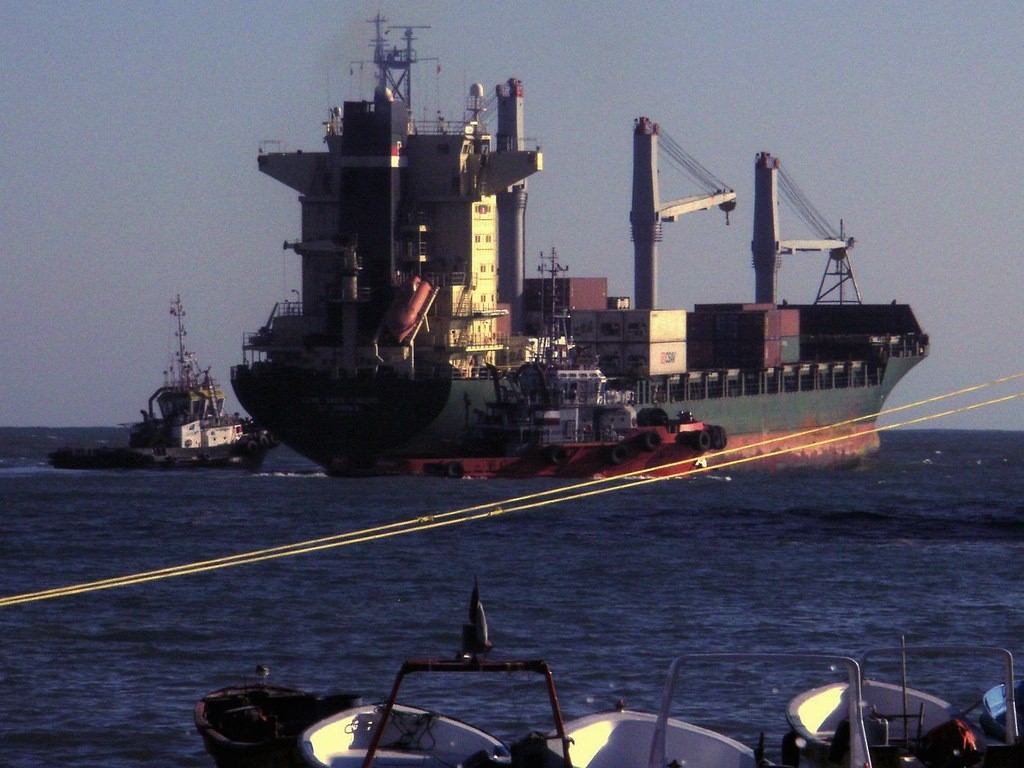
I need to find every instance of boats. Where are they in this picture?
[303,698,516,768]
[189,671,361,768]
[983,678,1024,745]
[550,701,781,768]
[784,675,987,754]
[369,252,726,480]
[54,291,280,474]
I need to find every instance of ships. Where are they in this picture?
[232,8,930,480]
